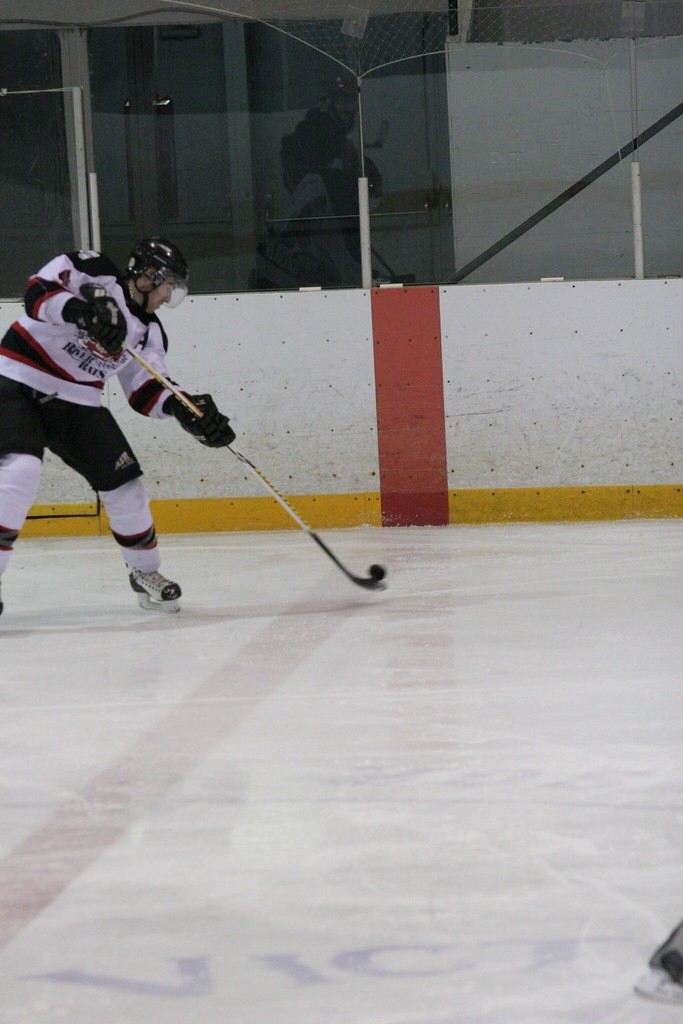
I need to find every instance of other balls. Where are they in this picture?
[369,564,385,577]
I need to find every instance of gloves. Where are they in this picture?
[162,391,236,447]
[62,296,127,355]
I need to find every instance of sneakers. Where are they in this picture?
[125,562,181,611]
[634,920,683,1006]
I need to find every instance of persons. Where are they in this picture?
[0,237,237,613]
[248,61,382,291]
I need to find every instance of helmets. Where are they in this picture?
[125,236,190,289]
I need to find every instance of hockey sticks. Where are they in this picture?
[89,314,385,588]
